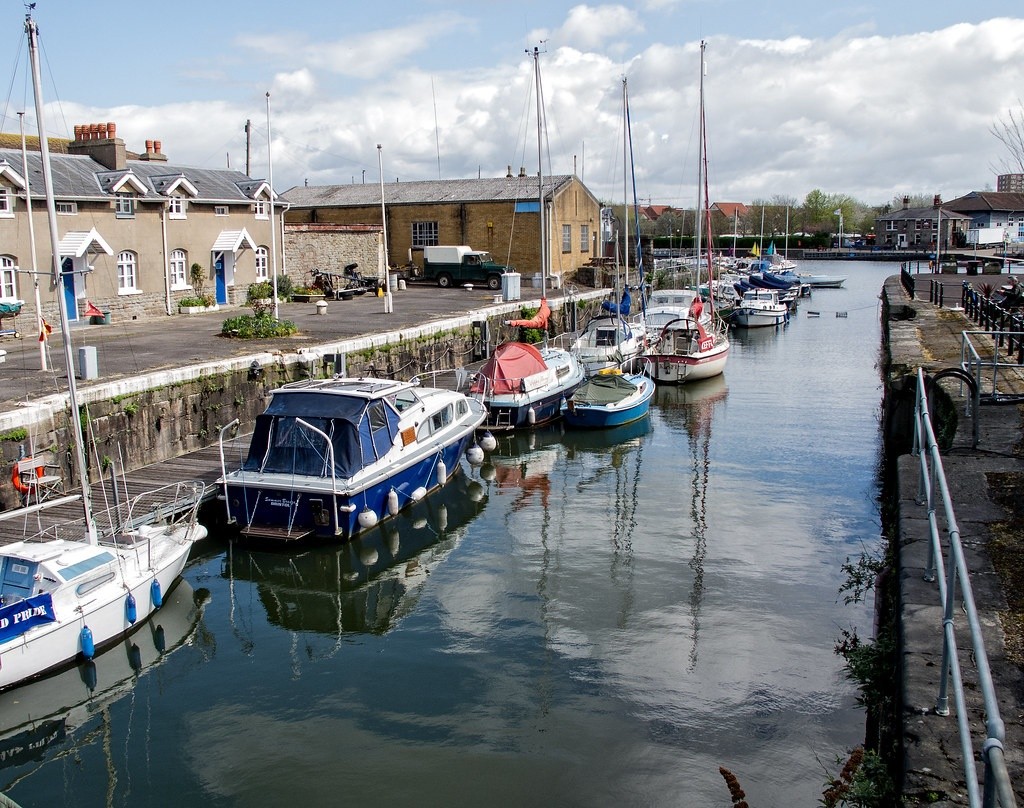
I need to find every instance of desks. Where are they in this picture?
[589,257,616,272]
[1001,285,1024,306]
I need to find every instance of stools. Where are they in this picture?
[583,263,598,268]
[603,262,620,270]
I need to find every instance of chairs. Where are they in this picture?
[17,455,67,508]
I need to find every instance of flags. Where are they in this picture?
[774,245,776,254]
[86,301,104,316]
[749,242,759,256]
[834,208,841,215]
[766,241,773,255]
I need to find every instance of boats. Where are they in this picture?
[560,356,658,427]
[632,287,711,337]
[695,252,848,329]
[211,374,490,544]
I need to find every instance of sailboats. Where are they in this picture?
[1,0,208,690]
[469,45,586,430]
[640,37,730,380]
[569,73,660,378]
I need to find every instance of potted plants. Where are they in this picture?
[248,274,293,305]
[179,263,220,314]
[291,285,326,303]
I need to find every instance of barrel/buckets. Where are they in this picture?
[95,311,111,325]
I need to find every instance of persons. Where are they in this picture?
[997,275,1024,312]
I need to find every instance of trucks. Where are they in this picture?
[423,246,516,290]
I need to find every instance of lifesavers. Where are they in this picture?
[11,457,45,494]
